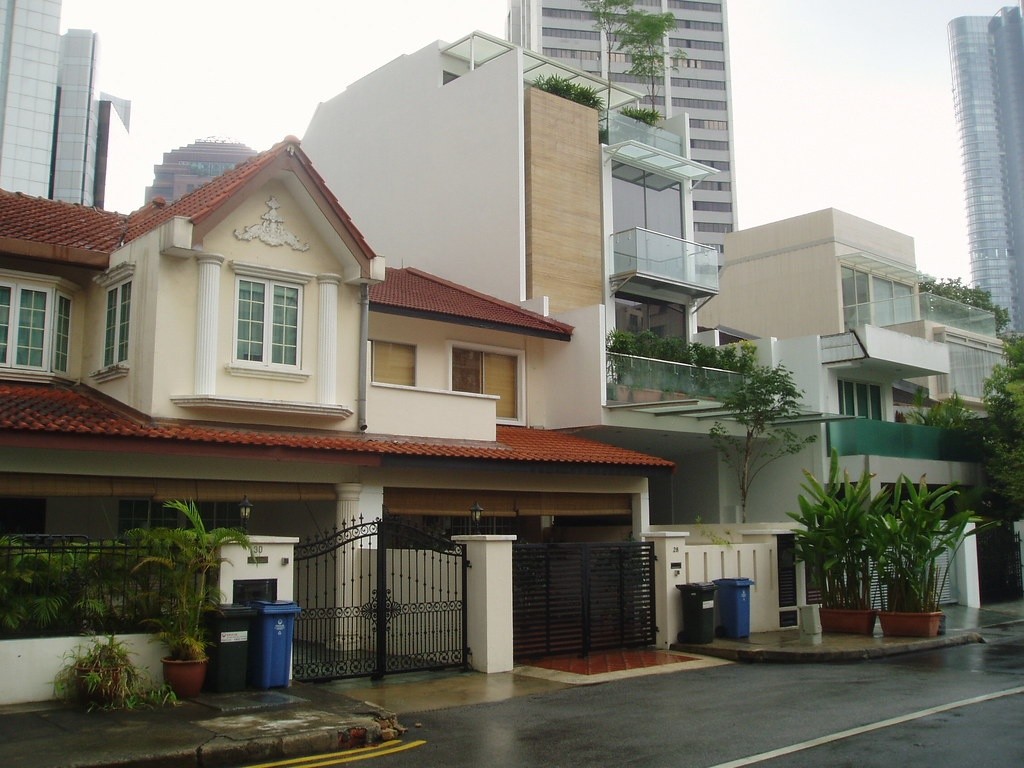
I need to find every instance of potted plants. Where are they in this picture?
[604,328,759,406]
[784,447,1005,638]
[130,498,256,697]
[55,627,187,712]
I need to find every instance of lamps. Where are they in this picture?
[469,500,484,534]
[238,494,254,533]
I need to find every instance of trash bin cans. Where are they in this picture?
[712,577,755,640]
[204,605,257,695]
[675,582,722,644]
[248,600,303,691]
